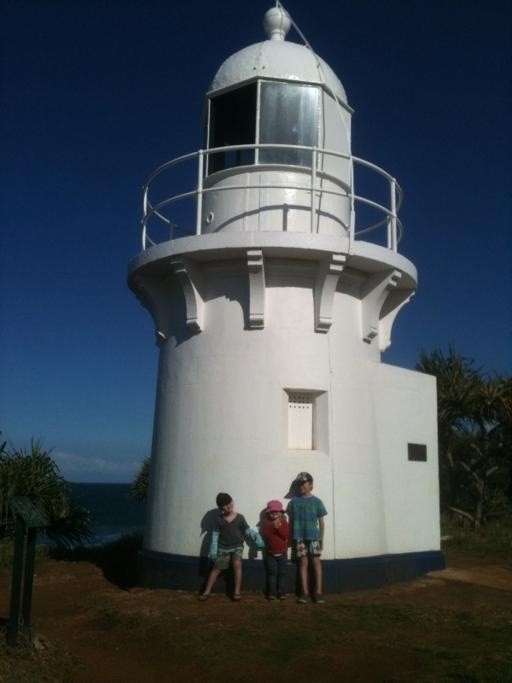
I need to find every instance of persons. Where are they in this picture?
[261,500,292,600]
[287,470,328,605]
[196,491,265,600]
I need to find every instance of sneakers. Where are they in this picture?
[268,595,286,600]
[297,593,325,604]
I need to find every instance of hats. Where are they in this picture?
[217,493,231,506]
[295,472,313,483]
[265,500,286,512]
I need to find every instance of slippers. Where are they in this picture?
[199,593,209,601]
[233,593,241,600]
